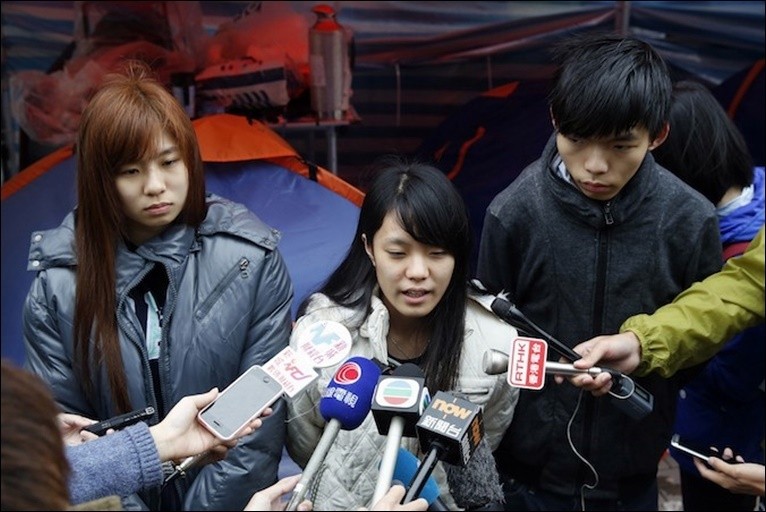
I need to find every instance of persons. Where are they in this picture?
[554,224,766,512]
[473,28,723,512]
[285,162,521,511]
[649,80,766,512]
[21,61,293,512]
[0,357,429,512]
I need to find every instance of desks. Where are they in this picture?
[266,101,362,177]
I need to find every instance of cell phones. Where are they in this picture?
[79,406,155,442]
[669,433,743,465]
[197,364,285,441]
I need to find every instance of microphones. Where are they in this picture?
[491,297,653,421]
[482,337,623,390]
[159,321,485,512]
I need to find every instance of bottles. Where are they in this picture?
[307,5,345,121]
